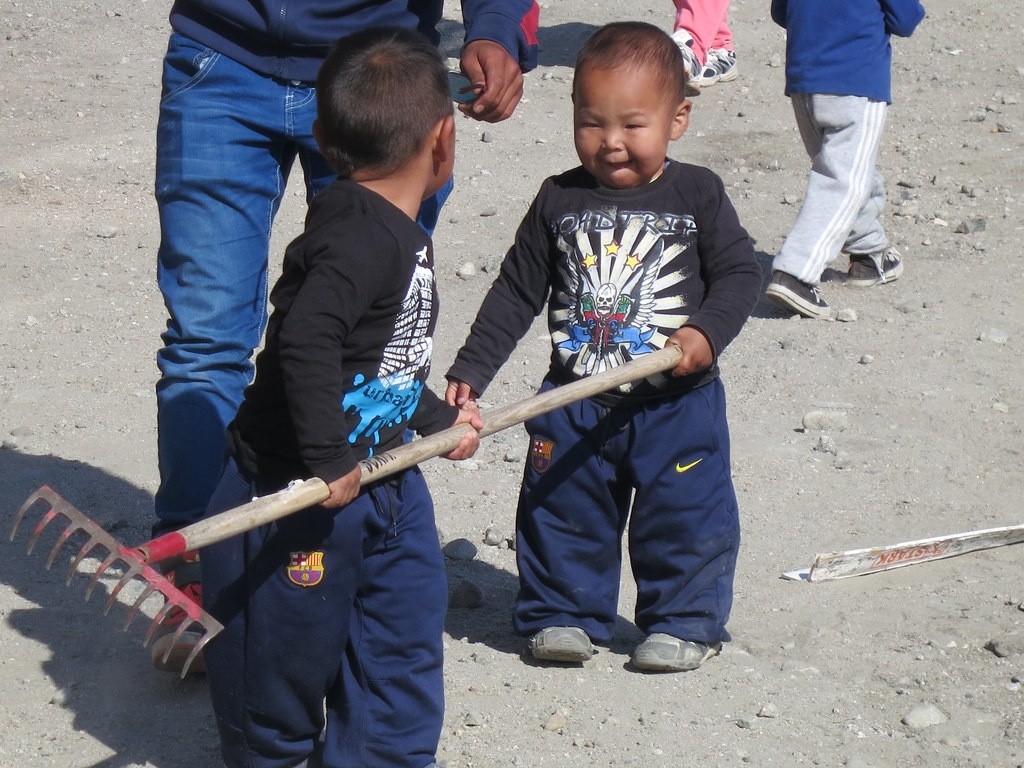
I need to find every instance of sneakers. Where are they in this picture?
[766,270,831,318]
[150,625,204,671]
[632,632,723,673]
[845,254,904,289]
[529,626,592,662]
[673,41,739,86]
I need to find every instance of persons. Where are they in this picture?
[661,0,741,88]
[194,23,480,768]
[767,0,928,322]
[439,21,765,671]
[152,0,539,676]
